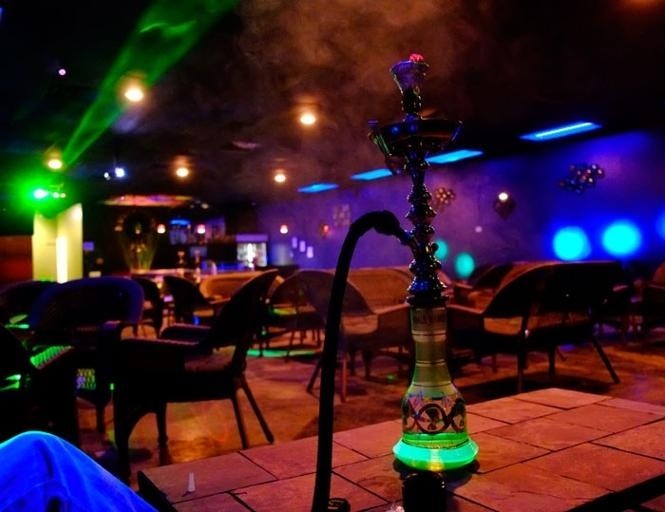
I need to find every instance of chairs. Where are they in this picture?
[3,278,58,338]
[445,259,625,397]
[30,274,144,437]
[270,270,323,348]
[198,272,286,305]
[0,326,75,445]
[109,268,276,462]
[293,268,416,402]
[163,275,229,321]
[348,268,416,380]
[471,259,559,376]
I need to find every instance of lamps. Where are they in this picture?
[303,53,482,512]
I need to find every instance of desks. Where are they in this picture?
[133,386,665,510]
